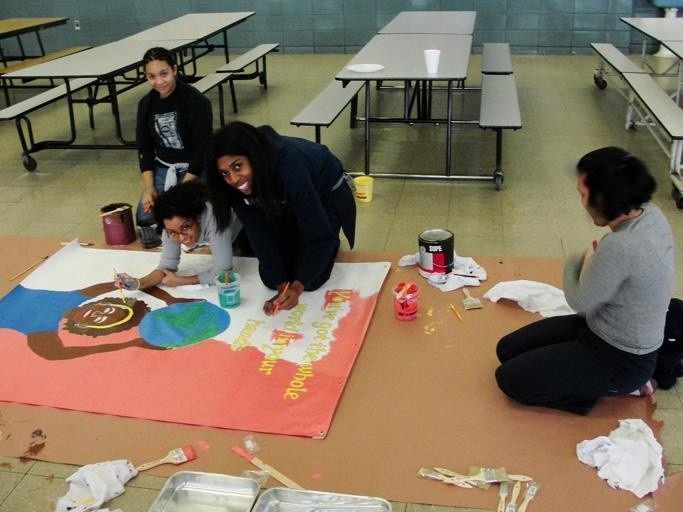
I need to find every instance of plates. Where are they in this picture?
[346,64,385,75]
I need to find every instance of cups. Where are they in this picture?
[424,49,441,74]
[391,283,419,321]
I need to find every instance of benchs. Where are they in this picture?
[290,76,366,145]
[1,11,280,171]
[621,72,683,177]
[475,74,522,190]
[481,41,513,75]
[589,41,649,132]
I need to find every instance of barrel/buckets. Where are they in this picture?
[353,176,374,203]
[392,278,420,320]
[101,202,137,246]
[419,228,454,282]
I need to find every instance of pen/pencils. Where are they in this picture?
[144,192,158,214]
[450,303,464,322]
[272,281,290,318]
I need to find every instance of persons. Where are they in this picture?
[208,121,356,317]
[499,147,676,417]
[136,46,212,228]
[112,183,242,291]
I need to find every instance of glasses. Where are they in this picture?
[166,217,194,239]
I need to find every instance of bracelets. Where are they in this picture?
[135,277,141,292]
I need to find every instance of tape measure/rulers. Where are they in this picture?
[230,445,305,490]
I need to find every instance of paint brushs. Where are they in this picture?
[113,268,127,305]
[10,256,49,281]
[60,242,95,246]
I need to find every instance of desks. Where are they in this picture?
[335,33,473,177]
[375,11,477,122]
[618,15,683,107]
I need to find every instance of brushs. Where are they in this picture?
[462,288,482,310]
[137,445,197,471]
[100,205,130,217]
[416,467,541,512]
